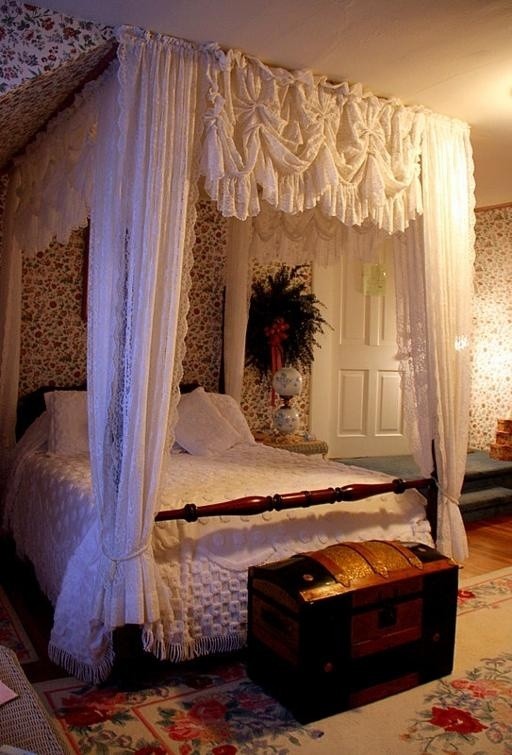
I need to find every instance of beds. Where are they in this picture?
[0,385,465,689]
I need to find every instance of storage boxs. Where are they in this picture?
[247,540,464,723]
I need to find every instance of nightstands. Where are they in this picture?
[252,432,328,460]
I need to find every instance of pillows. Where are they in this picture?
[43,387,252,458]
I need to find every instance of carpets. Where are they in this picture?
[33,565,512,755]
[0,590,38,664]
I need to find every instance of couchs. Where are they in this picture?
[0,644,77,755]
